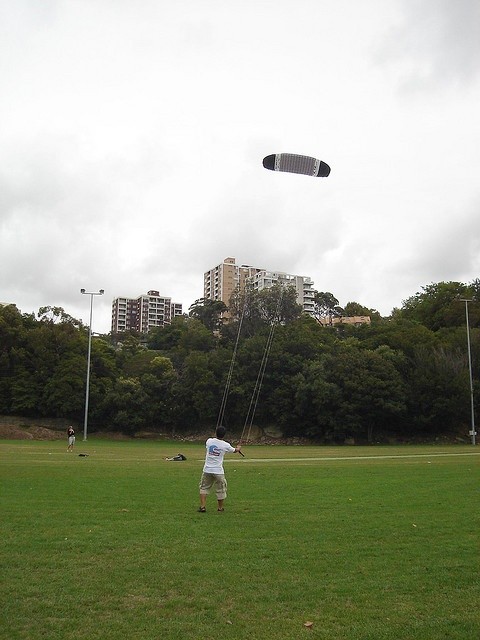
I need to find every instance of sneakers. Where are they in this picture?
[67,450,68,453]
[71,450,72,452]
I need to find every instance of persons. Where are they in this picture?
[165,454,185,460]
[66,426,75,452]
[199,426,240,513]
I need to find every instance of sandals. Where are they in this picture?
[197,507,206,512]
[218,508,224,511]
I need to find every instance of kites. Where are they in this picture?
[263,153,331,178]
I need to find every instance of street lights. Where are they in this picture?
[458,295,477,445]
[81,289,104,440]
